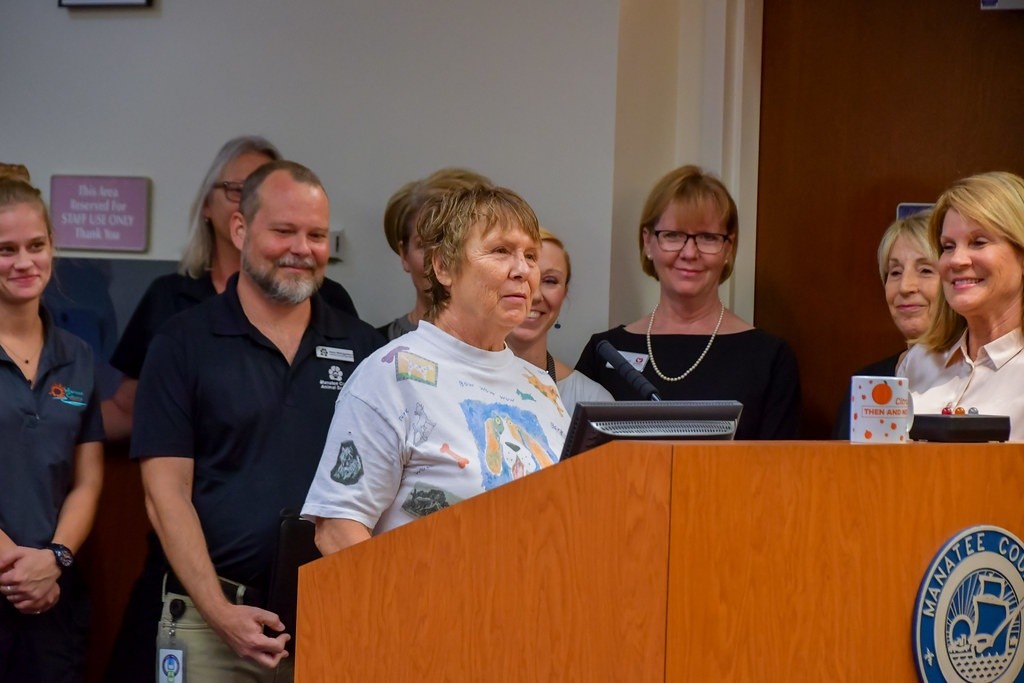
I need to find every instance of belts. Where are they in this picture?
[166,573,248,609]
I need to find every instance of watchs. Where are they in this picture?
[47,543,73,567]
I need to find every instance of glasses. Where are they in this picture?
[651,229,730,254]
[212,179,241,201]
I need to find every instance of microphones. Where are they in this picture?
[597,340,661,401]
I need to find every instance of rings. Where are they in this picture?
[7,586,10,591]
[35,611,40,614]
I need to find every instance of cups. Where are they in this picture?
[851,375,914,444]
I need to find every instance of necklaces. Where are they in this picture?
[646,303,723,381]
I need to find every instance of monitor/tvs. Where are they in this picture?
[559,400,743,462]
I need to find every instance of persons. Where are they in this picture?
[0,135,1024,683]
[575,167,797,441]
